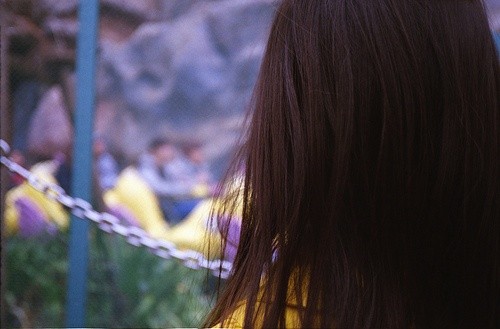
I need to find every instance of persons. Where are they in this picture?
[195,2,500,329]
[1,0,221,211]
